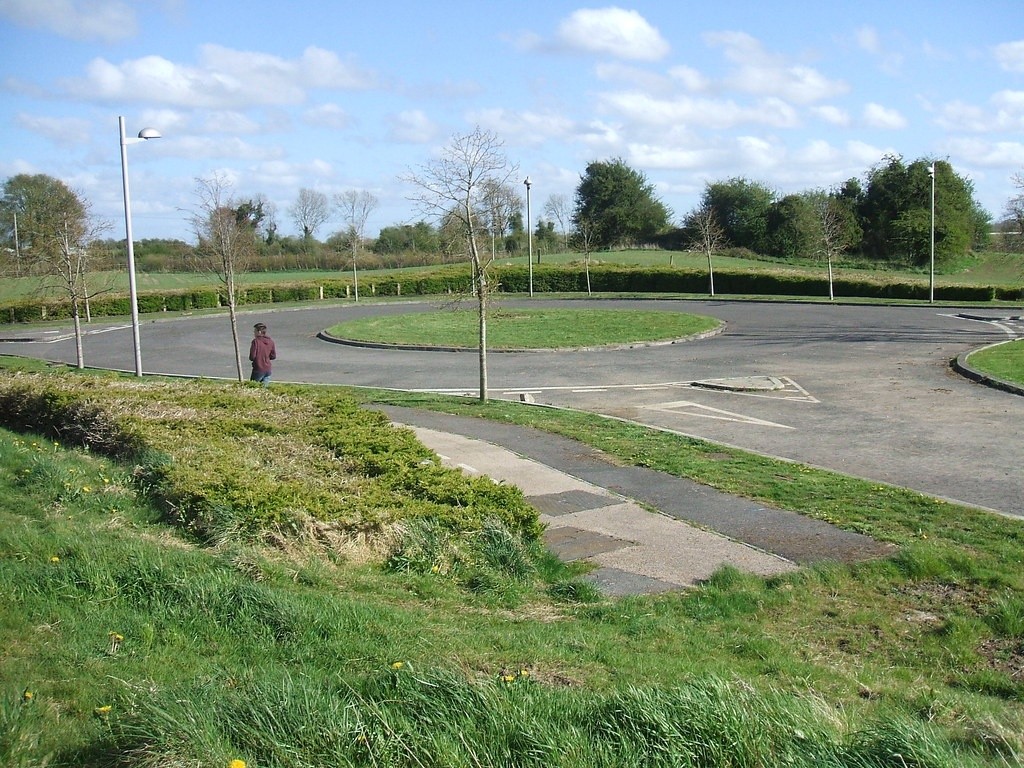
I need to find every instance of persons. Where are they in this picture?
[248,322,277,388]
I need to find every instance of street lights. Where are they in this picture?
[523,175,534,298]
[926,163,937,303]
[118,116,163,376]
